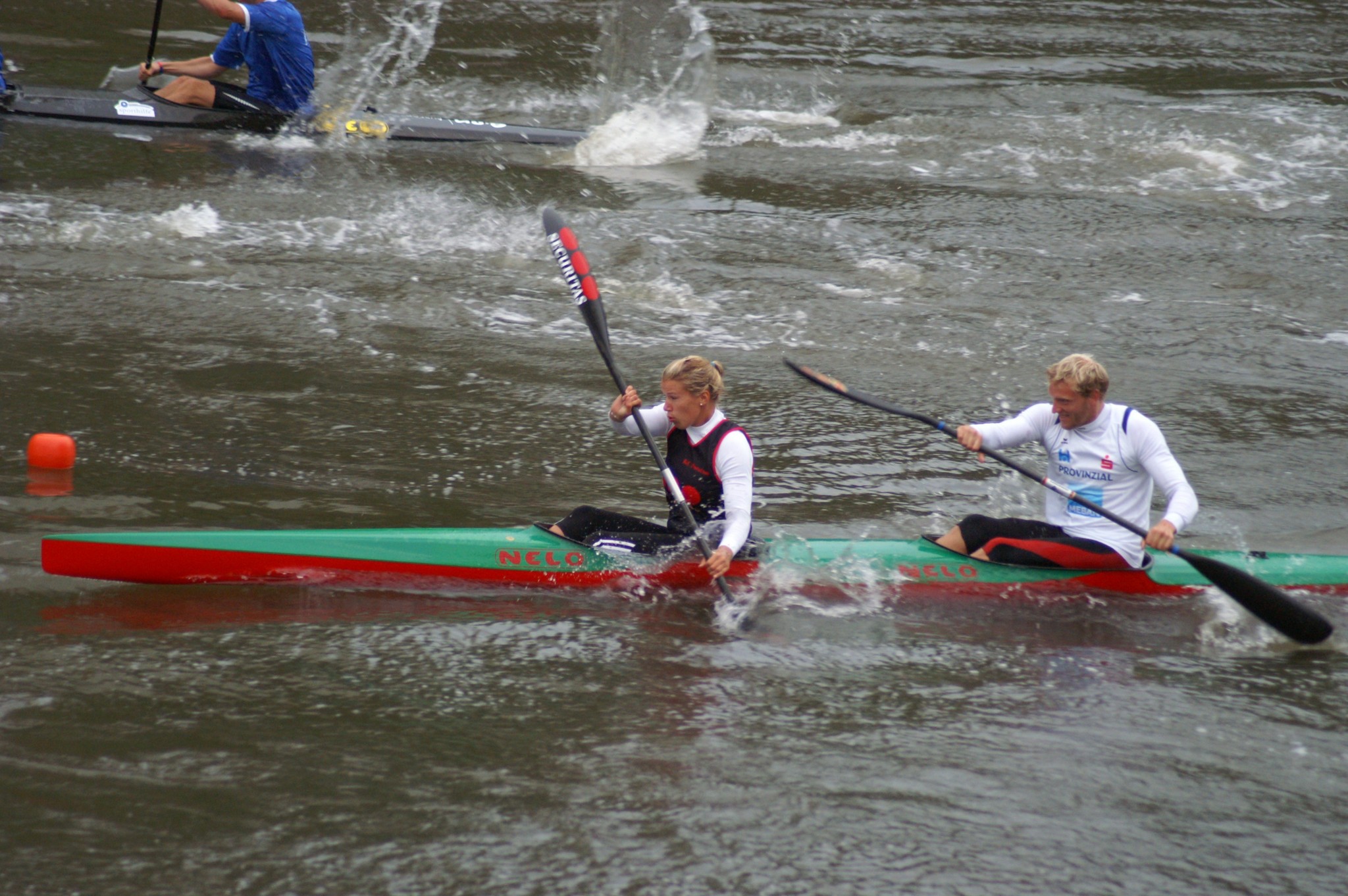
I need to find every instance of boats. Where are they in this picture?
[0,68,592,146]
[42,520,1348,607]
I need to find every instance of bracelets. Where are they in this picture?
[156,60,164,73]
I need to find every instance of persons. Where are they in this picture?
[138,1,316,116]
[548,356,753,579]
[936,354,1198,570]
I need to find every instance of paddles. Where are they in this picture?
[141,0,164,87]
[540,207,736,604]
[782,355,1336,646]
